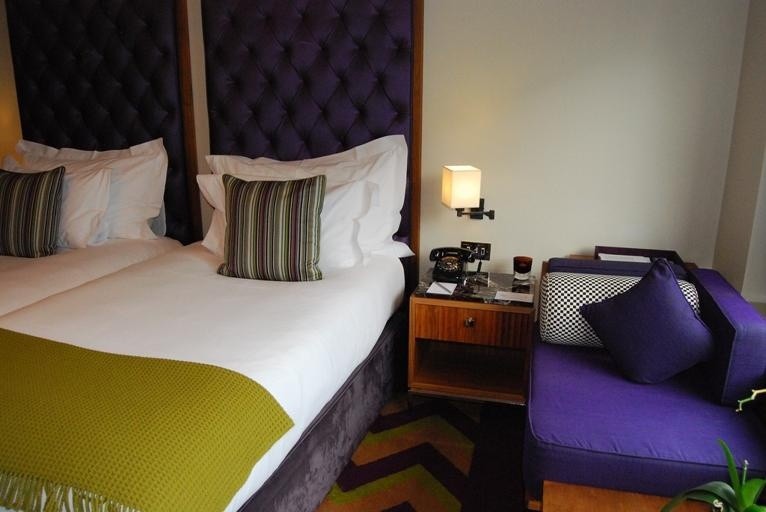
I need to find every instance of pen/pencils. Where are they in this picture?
[435,280,452,294]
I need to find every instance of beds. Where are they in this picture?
[0,0,425,512]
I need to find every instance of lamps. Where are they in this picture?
[441,164,496,222]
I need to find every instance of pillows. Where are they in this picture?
[580,258,721,386]
[0,166,66,258]
[539,269,702,347]
[15,137,168,240]
[205,134,417,258]
[216,174,326,282]
[2,155,118,249]
[195,174,370,272]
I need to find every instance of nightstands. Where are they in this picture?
[407,268,536,406]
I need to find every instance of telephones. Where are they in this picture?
[430,248,476,285]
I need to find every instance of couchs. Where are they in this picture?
[523,258,766,512]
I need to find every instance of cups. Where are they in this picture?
[514,256,533,281]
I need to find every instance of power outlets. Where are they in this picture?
[461,241,491,261]
